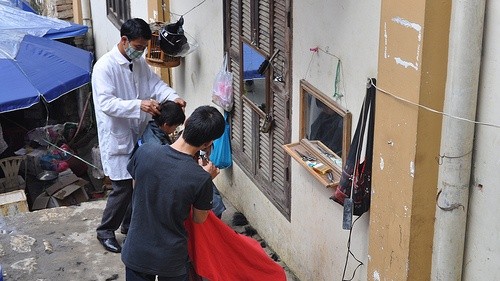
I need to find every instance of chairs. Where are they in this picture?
[0,156,27,193]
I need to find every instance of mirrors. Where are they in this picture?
[299,79,352,177]
[238,35,271,121]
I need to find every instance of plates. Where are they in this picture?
[36,171,59,181]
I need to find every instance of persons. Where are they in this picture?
[92,18,187,252]
[310,98,343,158]
[121,100,226,281]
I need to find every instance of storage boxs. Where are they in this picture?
[32,173,90,208]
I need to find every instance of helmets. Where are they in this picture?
[159,17,190,56]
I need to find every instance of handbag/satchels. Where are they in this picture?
[209,110,232,169]
[212,52,233,112]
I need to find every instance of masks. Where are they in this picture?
[123,43,143,60]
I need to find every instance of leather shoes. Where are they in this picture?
[97,235,122,253]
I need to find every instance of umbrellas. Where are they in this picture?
[0,0,94,114]
